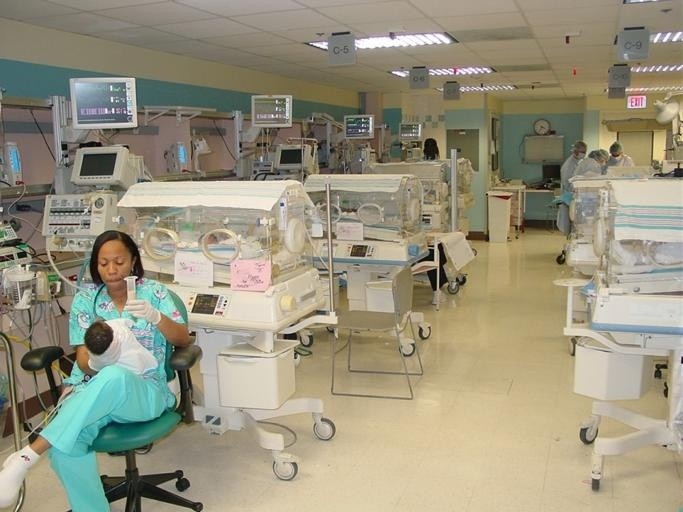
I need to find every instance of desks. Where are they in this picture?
[525,189,554,221]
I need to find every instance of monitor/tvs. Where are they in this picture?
[543,164,560,188]
[344,114,375,140]
[70,147,137,192]
[69,77,138,130]
[251,94,293,128]
[275,144,314,175]
[400,122,422,138]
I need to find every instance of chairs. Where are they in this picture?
[331,268,424,401]
[18,289,204,512]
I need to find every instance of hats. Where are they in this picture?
[609,142,622,153]
[596,150,610,161]
[574,141,586,150]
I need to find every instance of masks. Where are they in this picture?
[599,161,606,167]
[574,153,586,160]
[612,155,622,161]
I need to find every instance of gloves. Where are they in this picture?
[87,338,121,371]
[124,299,161,325]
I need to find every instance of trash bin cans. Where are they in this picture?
[486,191,513,243]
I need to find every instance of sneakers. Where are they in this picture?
[0,451,29,508]
[440,283,450,295]
[433,291,442,306]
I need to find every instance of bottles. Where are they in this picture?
[123,276,138,300]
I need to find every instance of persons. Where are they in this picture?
[412,242,451,303]
[84,319,113,355]
[0,232,189,512]
[611,143,633,168]
[424,137,440,159]
[554,141,587,237]
[573,149,609,177]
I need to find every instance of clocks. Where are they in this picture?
[533,119,551,136]
